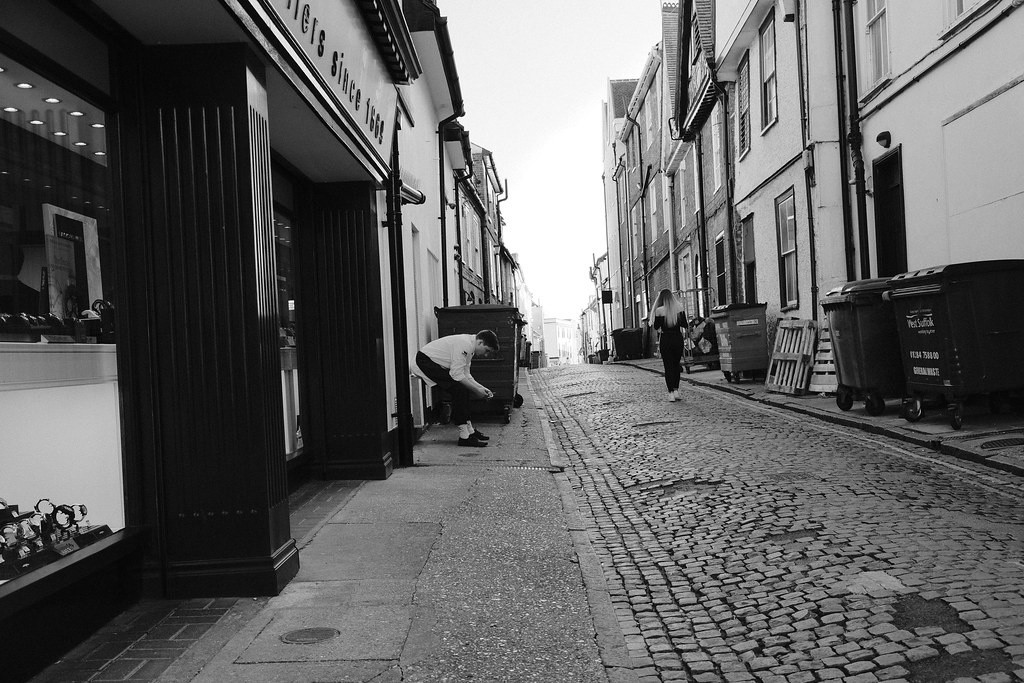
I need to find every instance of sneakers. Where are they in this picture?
[668,393,675,401]
[674,390,680,399]
[470,428,489,440]
[458,435,488,447]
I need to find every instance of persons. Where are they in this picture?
[648,289,688,401]
[415,330,499,447]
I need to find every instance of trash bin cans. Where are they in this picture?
[818,276,903,417]
[610,328,643,362]
[882,260,1024,424]
[433,305,532,424]
[710,301,769,382]
